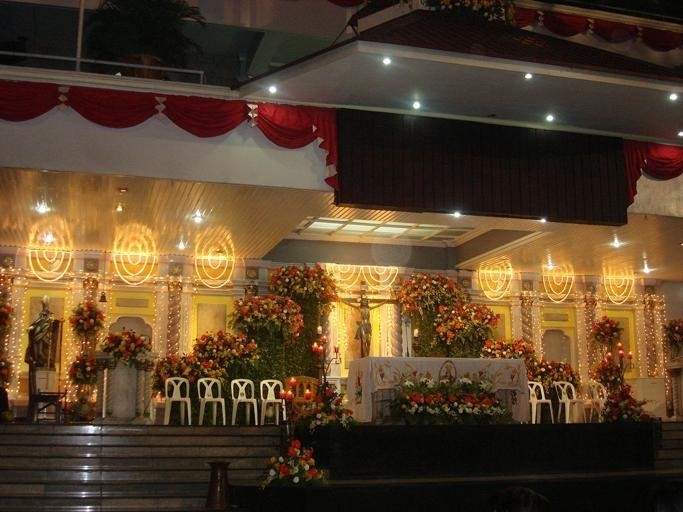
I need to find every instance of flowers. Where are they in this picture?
[261,434,335,491]
[156,262,351,431]
[395,273,582,388]
[67,298,151,386]
[591,315,654,422]
[398,374,506,426]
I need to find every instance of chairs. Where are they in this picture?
[26,358,66,423]
[525,376,608,424]
